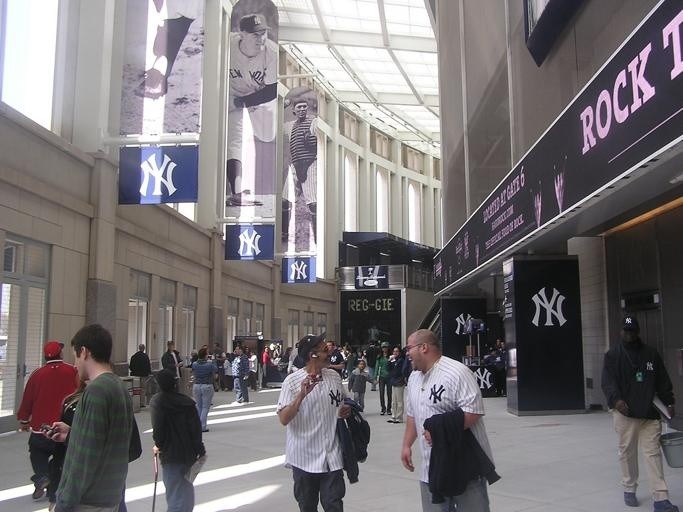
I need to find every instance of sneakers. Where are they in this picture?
[279,231,291,242]
[387,417,404,424]
[29,472,51,498]
[653,499,680,512]
[380,410,386,415]
[622,490,639,507]
[387,411,392,415]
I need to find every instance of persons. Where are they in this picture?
[150,369,204,512]
[19,324,133,511]
[401,329,496,512]
[129,344,151,407]
[261,342,415,423]
[133,0,205,98]
[277,332,352,512]
[161,341,258,403]
[192,347,216,433]
[226,13,279,206]
[290,100,318,184]
[485,338,506,396]
[602,315,680,512]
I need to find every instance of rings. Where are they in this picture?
[347,413,349,416]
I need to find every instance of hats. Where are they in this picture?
[619,316,642,331]
[237,12,274,33]
[44,342,64,357]
[381,341,389,348]
[297,331,328,360]
[157,369,180,392]
[292,97,309,108]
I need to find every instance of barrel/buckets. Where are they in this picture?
[659,418,683,468]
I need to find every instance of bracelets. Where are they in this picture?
[667,405,675,408]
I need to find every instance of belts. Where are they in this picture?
[195,382,212,385]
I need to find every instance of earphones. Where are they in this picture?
[312,354,319,358]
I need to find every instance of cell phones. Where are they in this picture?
[40,424,58,436]
